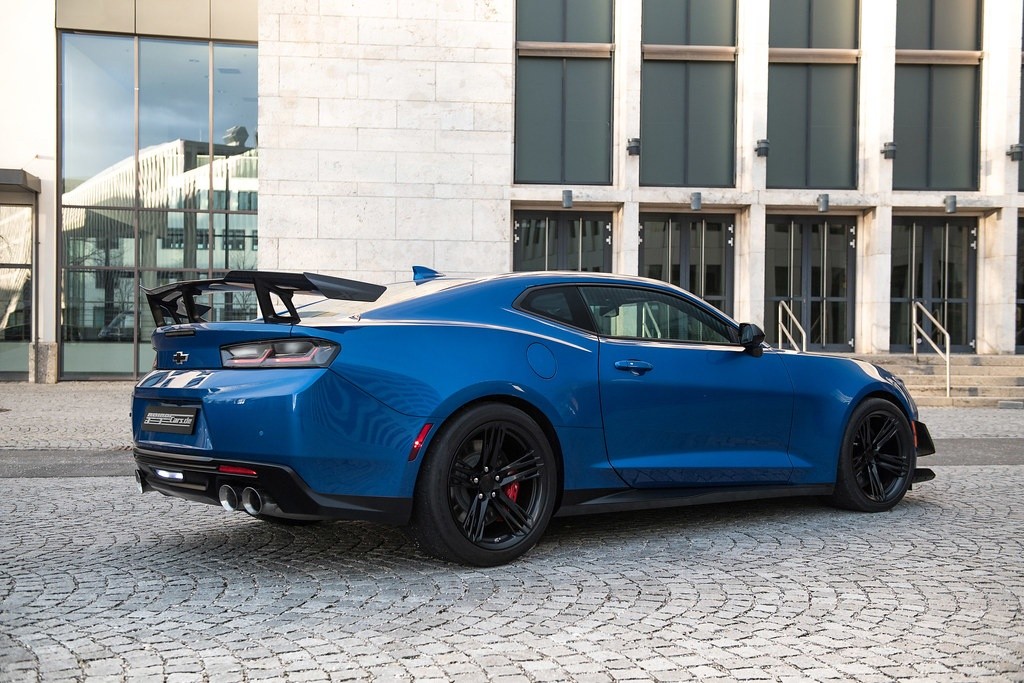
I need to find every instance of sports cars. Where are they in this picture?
[127,264,939,567]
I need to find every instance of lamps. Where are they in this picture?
[816,193,830,212]
[755,139,769,158]
[691,192,701,211]
[562,190,573,209]
[1007,144,1023,160]
[944,196,958,215]
[881,141,897,159]
[628,137,641,157]
